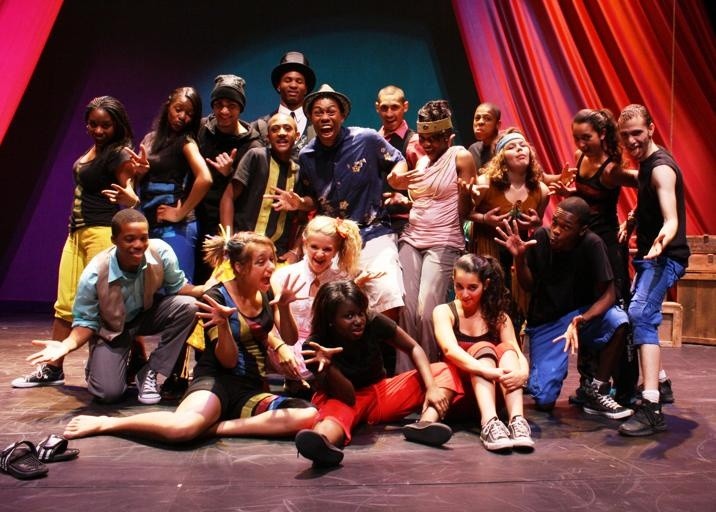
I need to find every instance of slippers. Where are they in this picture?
[0,440,49,478]
[35,433,81,463]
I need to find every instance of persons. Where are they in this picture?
[432,252,536,452]
[132,86,215,397]
[548,105,644,410]
[23,206,224,406]
[493,196,635,420]
[466,102,502,175]
[617,103,693,437]
[179,73,261,286]
[395,99,478,375]
[269,214,387,401]
[220,109,310,262]
[10,95,142,388]
[294,280,467,466]
[249,50,317,148]
[461,127,550,351]
[298,82,425,327]
[62,230,322,443]
[374,84,427,171]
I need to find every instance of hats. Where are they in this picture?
[302,83,352,121]
[210,74,248,114]
[271,51,317,96]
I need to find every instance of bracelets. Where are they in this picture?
[272,341,288,354]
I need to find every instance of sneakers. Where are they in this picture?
[12,363,65,388]
[295,429,344,464]
[402,420,453,447]
[480,376,675,451]
[134,360,162,405]
